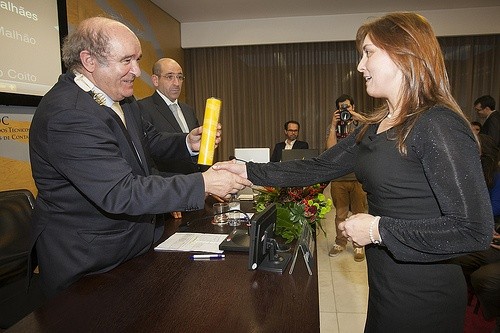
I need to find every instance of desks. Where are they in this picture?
[3,186,320,333]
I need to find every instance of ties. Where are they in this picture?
[169,104,186,132]
[111,102,127,129]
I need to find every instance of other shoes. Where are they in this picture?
[354,247,365,263]
[329,243,344,257]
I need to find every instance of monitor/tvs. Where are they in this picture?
[282,149,320,162]
[248,203,293,272]
[234,148,270,163]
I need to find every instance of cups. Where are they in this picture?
[213,203,228,223]
[227,202,241,227]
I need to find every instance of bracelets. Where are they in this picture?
[369,215,381,244]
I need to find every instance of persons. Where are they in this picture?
[26,17,255,289]
[270,121,308,162]
[213,12,495,333]
[137,58,206,218]
[470,95,500,318]
[326,94,369,263]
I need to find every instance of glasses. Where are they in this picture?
[154,74,186,83]
[286,130,298,132]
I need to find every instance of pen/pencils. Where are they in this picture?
[189,253,226,259]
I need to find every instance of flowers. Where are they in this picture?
[250,181,334,245]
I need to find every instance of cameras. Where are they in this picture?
[336,104,353,125]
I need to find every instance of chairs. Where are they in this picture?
[0,188,36,333]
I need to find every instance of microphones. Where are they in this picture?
[229,155,247,163]
[178,209,251,251]
